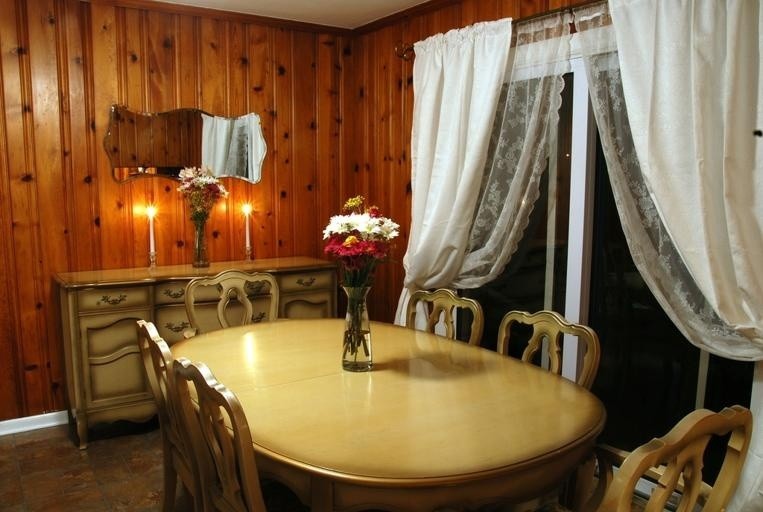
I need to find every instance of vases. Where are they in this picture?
[191,222,211,267]
[341,287,373,373]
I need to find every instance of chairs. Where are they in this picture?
[171,356,313,512]
[184,269,280,336]
[496,309,602,512]
[406,287,486,345]
[536,404,754,511]
[134,319,204,512]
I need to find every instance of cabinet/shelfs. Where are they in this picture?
[53,254,341,451]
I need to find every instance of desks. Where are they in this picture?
[166,319,609,512]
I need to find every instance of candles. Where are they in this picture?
[245,213,250,248]
[148,208,155,253]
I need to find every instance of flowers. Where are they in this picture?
[322,195,401,363]
[176,167,230,261]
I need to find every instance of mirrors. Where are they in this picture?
[103,102,268,185]
